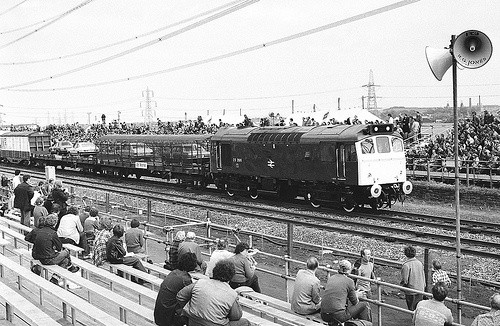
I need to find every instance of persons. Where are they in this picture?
[471,293,500,326]
[413,282,453,326]
[163,231,207,275]
[154,252,197,326]
[400,246,426,319]
[404,110,500,178]
[205,237,267,306]
[350,248,377,309]
[291,257,322,323]
[0,167,153,287]
[432,260,451,303]
[321,260,369,326]
[0,111,424,157]
[176,261,252,326]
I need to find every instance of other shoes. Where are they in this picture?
[255,299,268,305]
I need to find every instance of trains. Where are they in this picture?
[0,123,413,213]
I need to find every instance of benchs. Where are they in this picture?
[0,211,329,326]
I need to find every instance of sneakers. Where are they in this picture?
[48,268,82,288]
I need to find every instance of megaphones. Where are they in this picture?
[452,30,493,69]
[425,46,454,81]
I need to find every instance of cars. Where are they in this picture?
[161,143,210,164]
[115,142,153,162]
[49,141,99,157]
